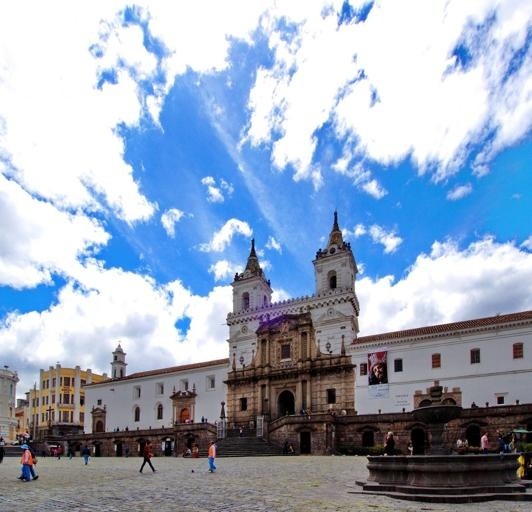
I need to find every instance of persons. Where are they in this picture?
[81,445,91,465]
[288,444,294,455]
[385,432,395,455]
[18,435,30,445]
[481,432,489,454]
[208,441,216,472]
[126,446,129,458]
[368,363,388,384]
[184,447,191,455]
[496,432,519,452]
[58,445,63,459]
[193,446,198,458]
[457,435,468,448]
[18,444,38,482]
[140,442,157,472]
[70,446,73,458]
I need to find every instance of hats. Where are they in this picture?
[20,443,29,450]
